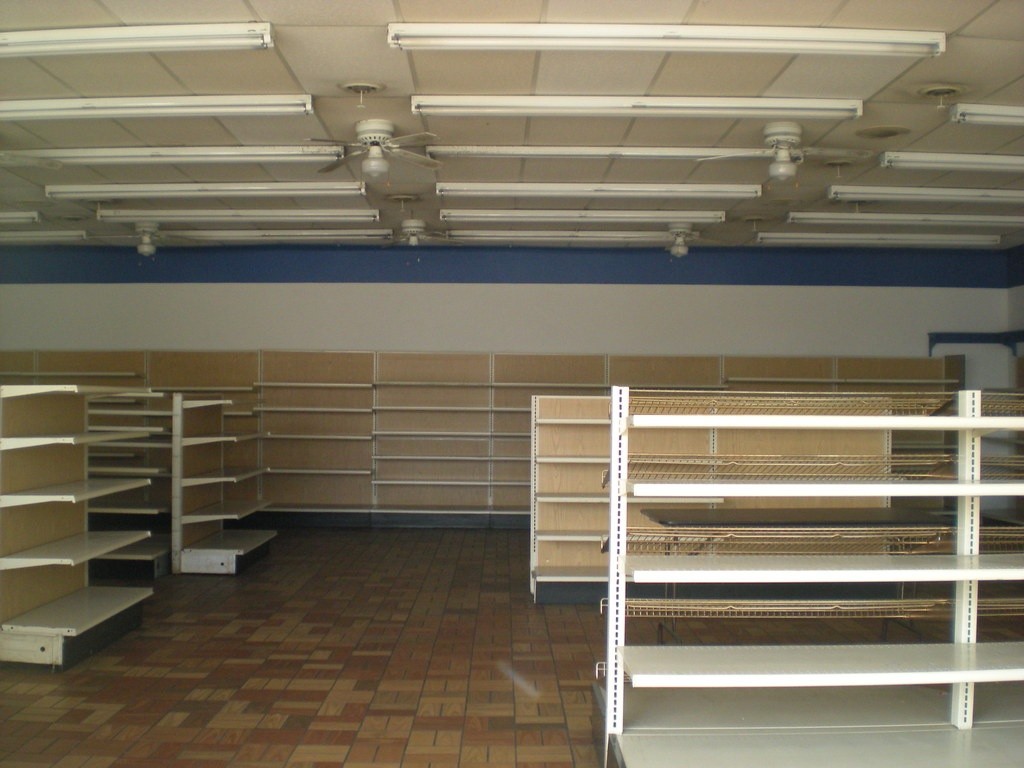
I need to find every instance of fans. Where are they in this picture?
[95,225,194,247]
[300,119,444,176]
[693,121,875,170]
[380,219,451,249]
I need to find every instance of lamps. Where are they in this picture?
[45,182,368,202]
[0,212,45,227]
[408,234,419,246]
[164,229,395,244]
[0,93,315,122]
[0,230,91,246]
[666,222,694,258]
[447,230,702,245]
[0,144,347,170]
[755,102,1024,247]
[361,144,389,178]
[138,237,155,256]
[411,93,862,120]
[438,210,726,224]
[387,22,949,60]
[437,182,764,201]
[0,22,277,59]
[96,209,381,223]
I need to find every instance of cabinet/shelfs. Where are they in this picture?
[87,390,171,585]
[145,348,260,525]
[171,393,275,575]
[491,353,607,529]
[253,350,373,531]
[722,355,836,394]
[36,350,147,393]
[596,386,1024,768]
[605,355,721,390]
[836,354,965,514]
[529,394,894,605]
[0,386,153,671]
[368,353,491,529]
